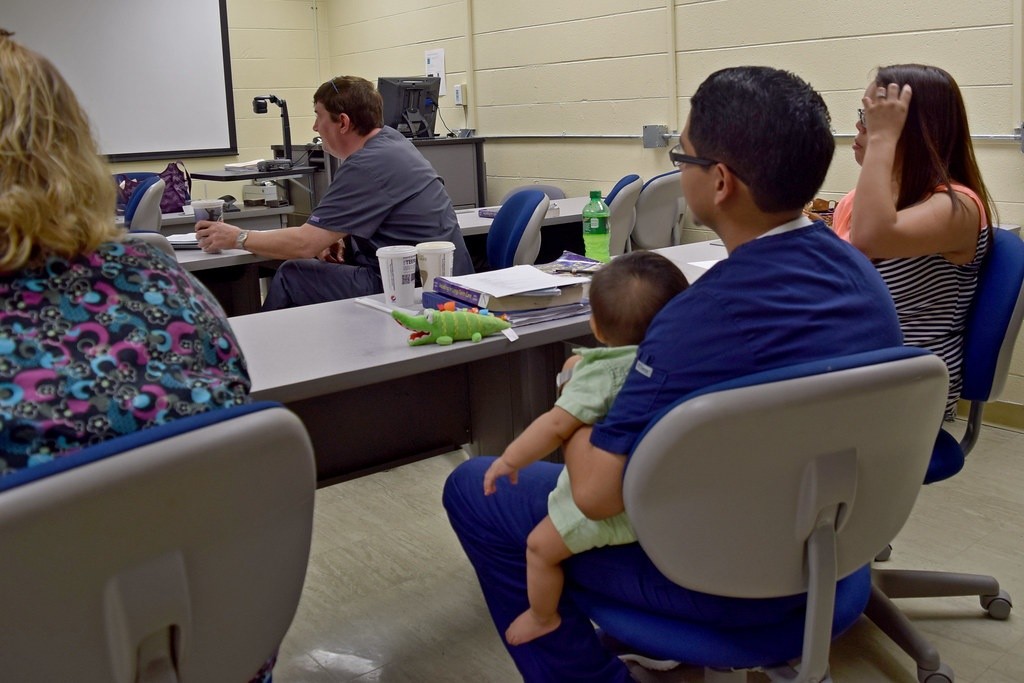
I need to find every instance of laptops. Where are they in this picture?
[166,234,200,249]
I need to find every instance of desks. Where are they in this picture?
[228,236,753,403]
[118,201,295,235]
[169,193,657,275]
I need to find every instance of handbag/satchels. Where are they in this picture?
[115,161,191,215]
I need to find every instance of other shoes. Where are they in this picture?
[593,627,679,670]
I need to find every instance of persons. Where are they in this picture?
[194,76,476,312]
[442,66,904,683]
[833,64,995,423]
[482,249,690,646]
[0,36,252,477]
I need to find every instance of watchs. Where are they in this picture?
[238,230,251,250]
[556,368,572,400]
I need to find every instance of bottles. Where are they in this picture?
[581,190,611,263]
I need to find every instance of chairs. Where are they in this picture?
[0,400,316,683]
[864,225,1024,683]
[585,347,948,683]
[124,175,165,230]
[486,170,687,269]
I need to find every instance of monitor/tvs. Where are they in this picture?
[378,77,441,138]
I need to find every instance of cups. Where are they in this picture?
[415,240,457,293]
[190,199,225,254]
[376,246,418,308]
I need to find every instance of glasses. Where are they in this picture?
[668,143,753,188]
[858,108,866,129]
